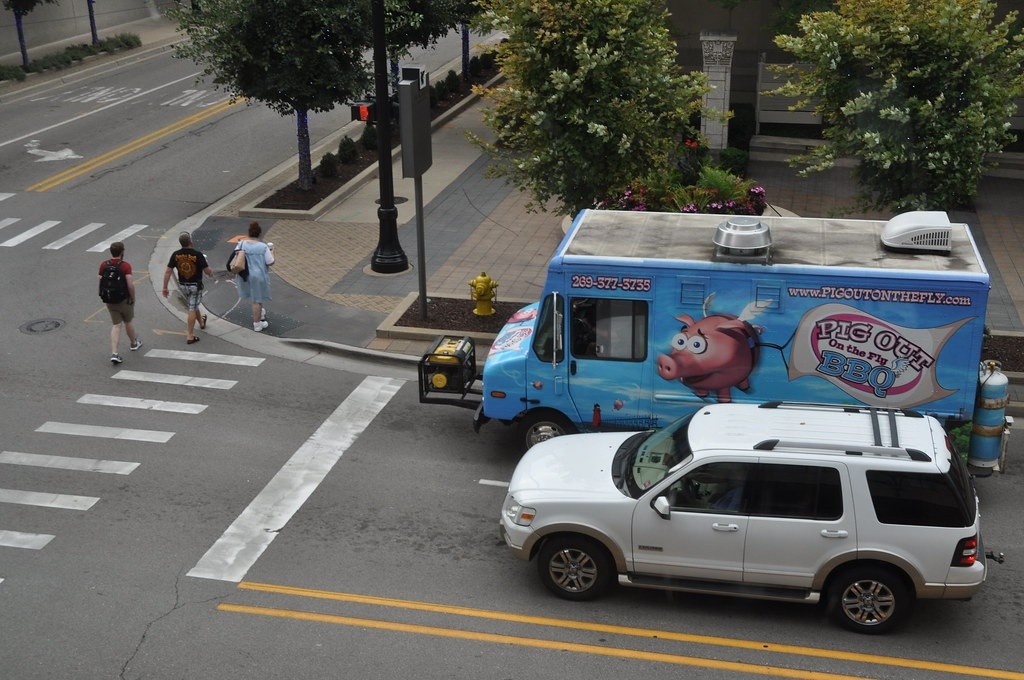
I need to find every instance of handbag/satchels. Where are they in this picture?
[230,240,245,273]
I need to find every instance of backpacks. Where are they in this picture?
[99,260,130,304]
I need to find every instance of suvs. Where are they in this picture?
[499,400,1006,637]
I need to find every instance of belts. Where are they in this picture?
[179,283,196,286]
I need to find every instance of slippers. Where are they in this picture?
[200,314,206,329]
[187,336,200,344]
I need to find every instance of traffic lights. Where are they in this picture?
[350,100,377,124]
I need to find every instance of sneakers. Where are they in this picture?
[261,308,266,320]
[253,321,268,331]
[110,353,123,363]
[130,336,142,350]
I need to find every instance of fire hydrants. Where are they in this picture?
[468,271,498,317]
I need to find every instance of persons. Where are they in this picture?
[98,242,143,363]
[163,234,213,344]
[234,222,275,332]
[712,473,747,510]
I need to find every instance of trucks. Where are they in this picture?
[418,208,1014,459]
